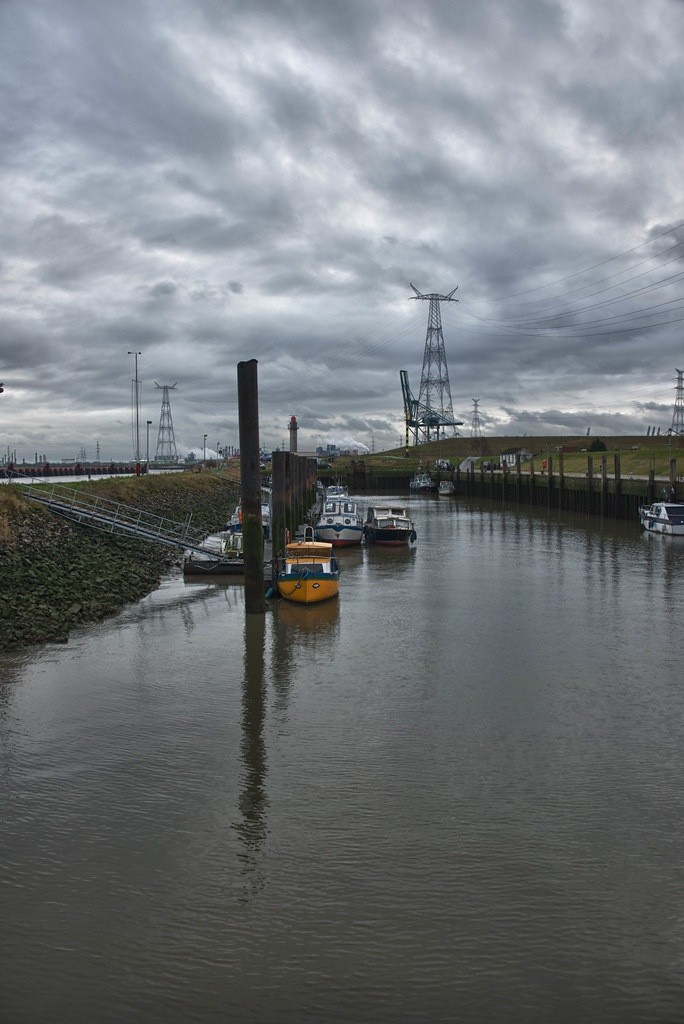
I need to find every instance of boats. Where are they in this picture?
[276,527,340,607]
[409,473,437,495]
[437,480,456,496]
[364,505,417,548]
[227,502,271,540]
[638,500,684,536]
[313,474,365,549]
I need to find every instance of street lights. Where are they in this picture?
[204,435,208,462]
[217,443,220,460]
[146,420,153,471]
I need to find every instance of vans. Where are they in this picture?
[434,459,450,471]
